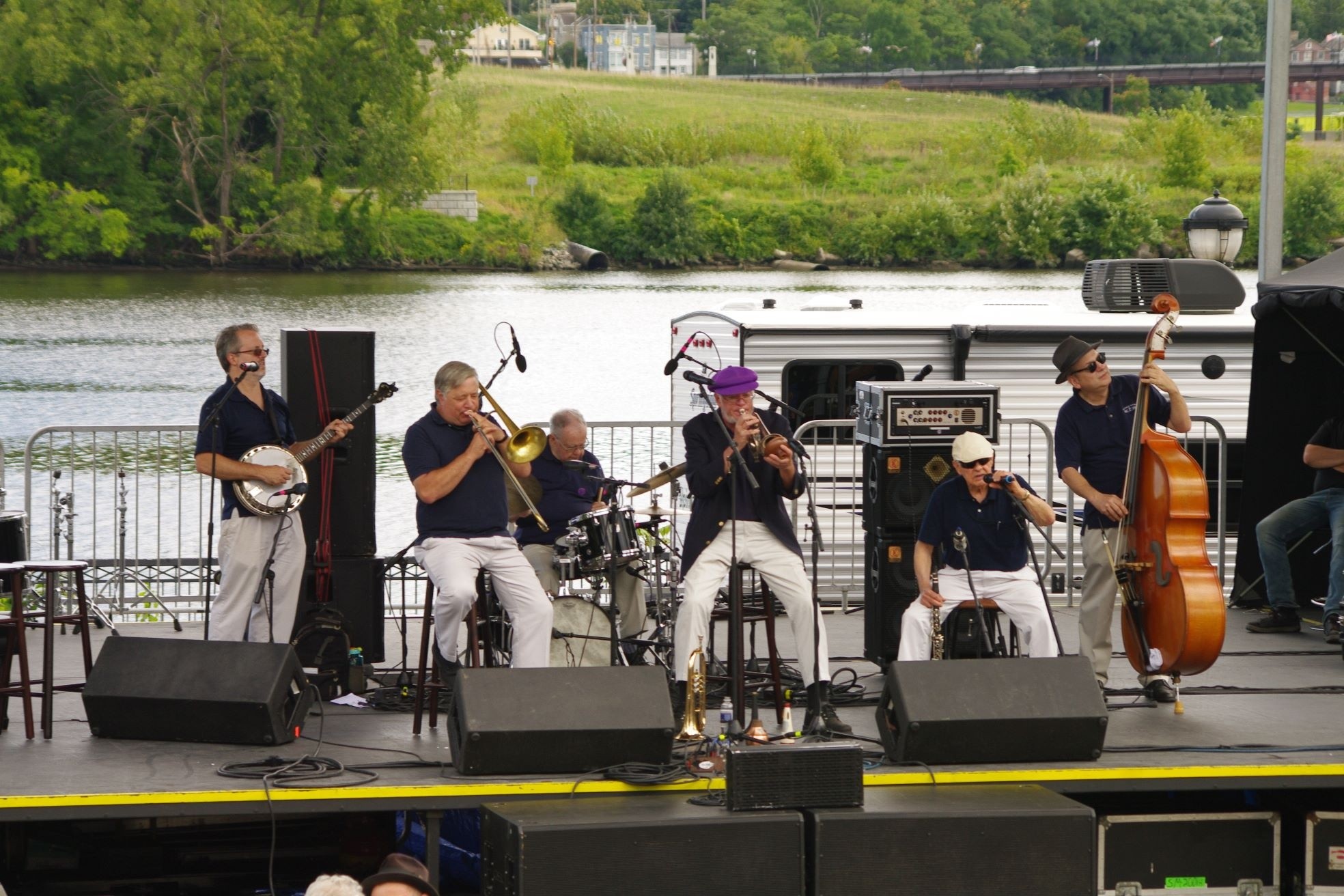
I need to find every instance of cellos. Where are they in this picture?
[1105,293,1227,714]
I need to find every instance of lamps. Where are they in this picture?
[1180,188,1251,270]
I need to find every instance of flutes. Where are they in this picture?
[929,563,947,660]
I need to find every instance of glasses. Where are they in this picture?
[232,348,269,357]
[1071,352,1106,376]
[956,457,990,469]
[553,433,589,451]
[721,394,755,404]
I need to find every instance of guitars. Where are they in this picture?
[232,380,399,516]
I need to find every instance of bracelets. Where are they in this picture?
[1019,487,1030,501]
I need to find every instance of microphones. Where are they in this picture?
[274,483,308,496]
[511,326,527,372]
[552,627,563,638]
[914,365,933,381]
[563,459,601,470]
[787,437,810,460]
[953,527,967,550]
[984,474,1015,484]
[240,361,260,372]
[664,333,696,375]
[683,371,715,385]
[626,566,646,581]
[634,519,667,528]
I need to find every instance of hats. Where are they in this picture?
[952,431,993,463]
[362,853,438,896]
[707,365,759,396]
[1052,335,1103,384]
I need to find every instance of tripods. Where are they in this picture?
[22,469,183,636]
[625,353,884,750]
[355,542,447,695]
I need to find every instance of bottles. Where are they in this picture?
[779,703,795,744]
[716,734,730,778]
[745,720,768,745]
[348,648,364,667]
[719,696,734,735]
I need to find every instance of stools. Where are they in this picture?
[0,563,36,739]
[705,562,784,740]
[943,598,1022,658]
[0,560,94,740]
[411,566,494,736]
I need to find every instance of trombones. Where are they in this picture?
[466,381,550,532]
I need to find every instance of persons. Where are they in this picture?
[898,431,1060,662]
[672,366,853,736]
[1052,336,1192,704]
[195,323,355,645]
[1245,415,1344,643]
[514,408,651,667]
[401,361,555,690]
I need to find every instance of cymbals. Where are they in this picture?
[632,505,693,515]
[626,461,688,498]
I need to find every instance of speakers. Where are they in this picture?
[862,531,995,669]
[860,442,961,539]
[82,636,315,746]
[808,783,1096,896]
[725,741,865,812]
[279,328,376,556]
[445,667,676,774]
[482,794,808,896]
[289,556,385,668]
[874,656,1109,766]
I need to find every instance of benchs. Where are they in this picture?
[1036,438,1247,583]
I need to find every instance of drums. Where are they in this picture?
[553,532,591,581]
[567,504,646,572]
[505,593,619,667]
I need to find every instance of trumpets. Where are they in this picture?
[673,634,708,742]
[739,407,785,463]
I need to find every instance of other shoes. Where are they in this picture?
[802,701,853,737]
[1245,606,1301,632]
[1324,614,1342,643]
[1147,679,1176,703]
[624,650,655,666]
